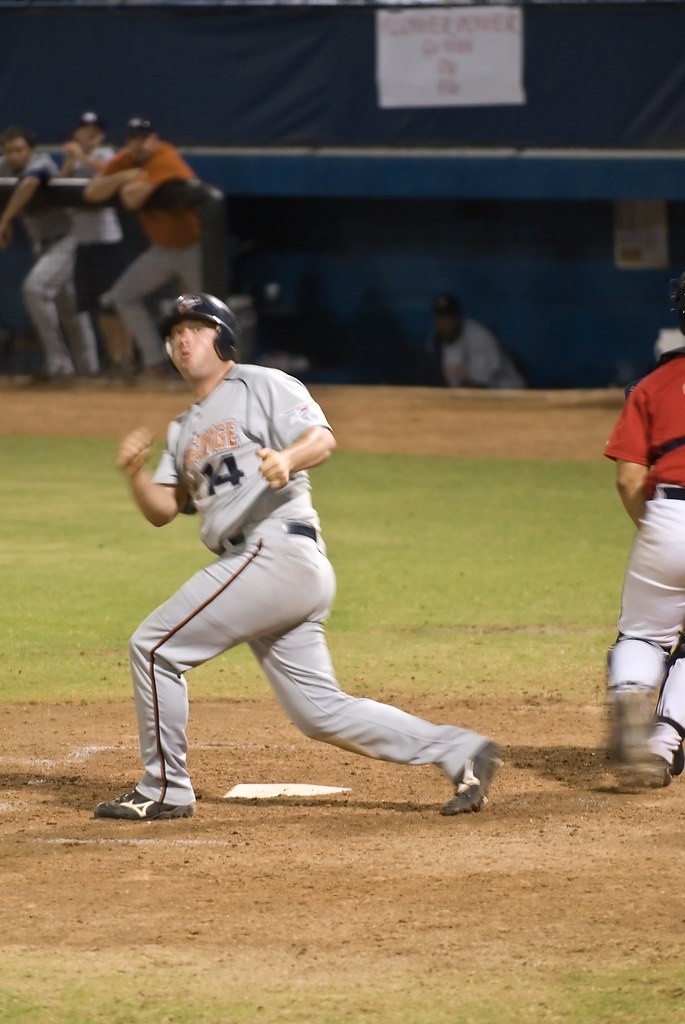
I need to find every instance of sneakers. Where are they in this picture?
[438,741,498,816]
[95,788,194,823]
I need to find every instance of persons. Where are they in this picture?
[593,272,685,790]
[94,289,504,824]
[1,108,314,391]
[431,296,526,394]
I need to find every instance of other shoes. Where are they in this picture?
[590,693,671,793]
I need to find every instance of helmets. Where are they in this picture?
[162,292,240,362]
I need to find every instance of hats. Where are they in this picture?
[128,112,156,137]
[68,110,103,136]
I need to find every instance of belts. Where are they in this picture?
[648,487,685,500]
[217,523,316,548]
